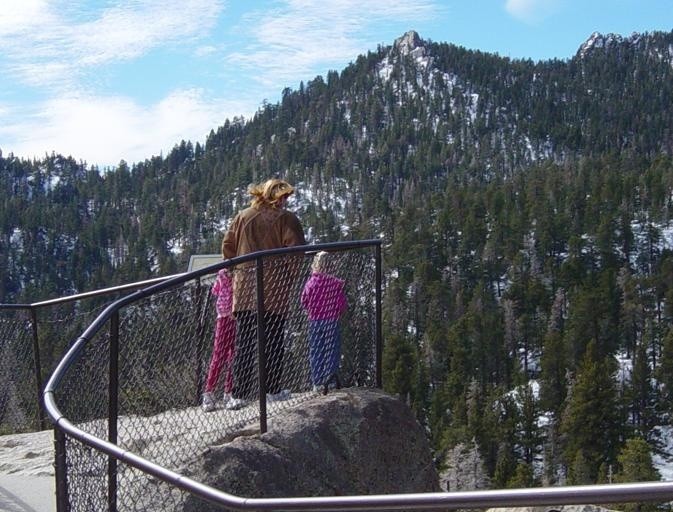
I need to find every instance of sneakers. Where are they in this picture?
[203,384,337,411]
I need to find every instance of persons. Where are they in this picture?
[300,250,349,396]
[222,179,306,409]
[202,265,237,413]
